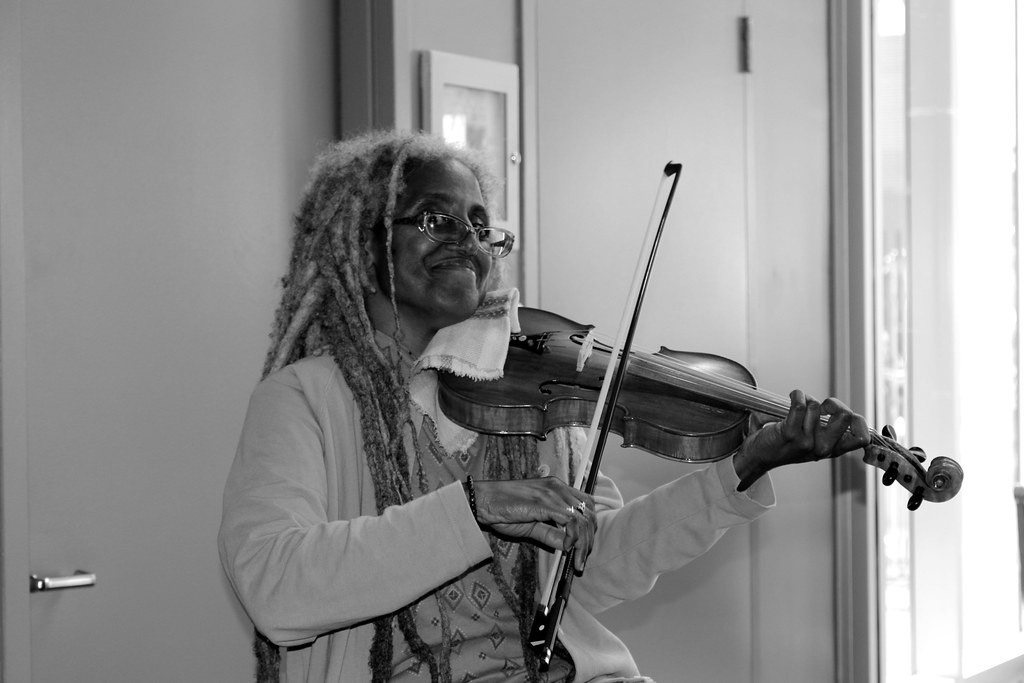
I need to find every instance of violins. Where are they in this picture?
[435,305,964,512]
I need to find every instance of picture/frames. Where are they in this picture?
[424,48,520,252]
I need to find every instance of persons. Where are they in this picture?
[215,131,869,681]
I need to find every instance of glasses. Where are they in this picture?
[379,210,516,258]
